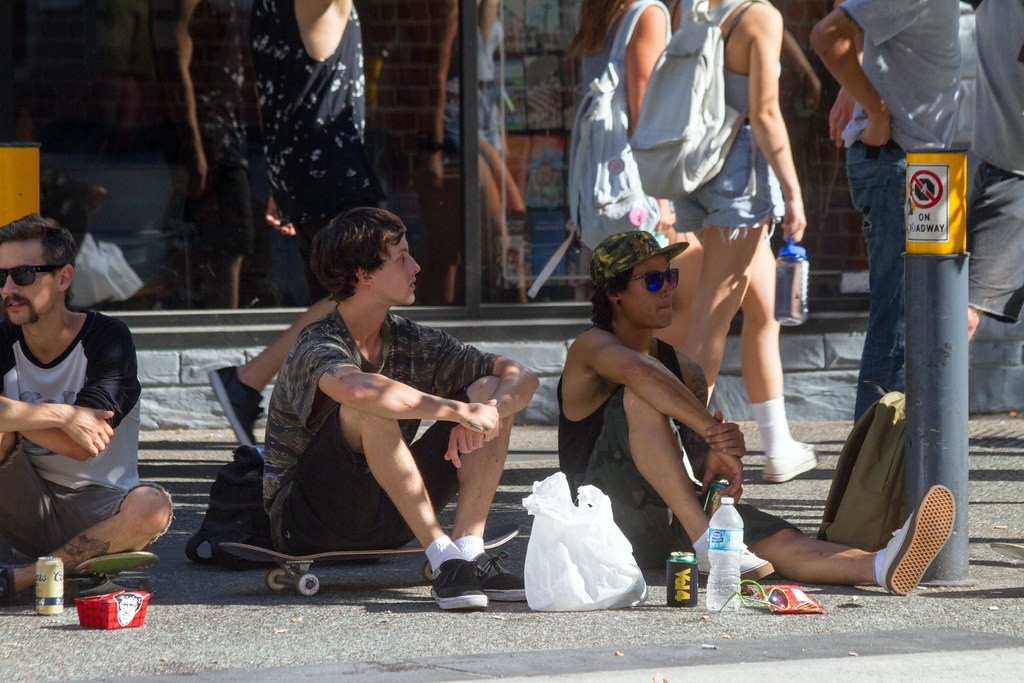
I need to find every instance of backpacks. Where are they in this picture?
[527,1,671,299]
[629,0,775,200]
[816,380,907,552]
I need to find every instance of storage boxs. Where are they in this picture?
[74,590,153,630]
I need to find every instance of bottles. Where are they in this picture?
[775,234,809,326]
[706,497,744,611]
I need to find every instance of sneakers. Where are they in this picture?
[472,551,526,602]
[875,484,956,595]
[208,365,265,447]
[431,559,489,610]
[696,541,775,586]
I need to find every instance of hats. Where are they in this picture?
[589,231,690,289]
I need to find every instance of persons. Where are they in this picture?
[165,0,258,309]
[210,0,388,449]
[573,0,1024,485]
[262,205,525,609]
[0,213,174,606]
[424,0,528,307]
[557,230,957,597]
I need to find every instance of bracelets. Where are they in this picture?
[427,141,444,155]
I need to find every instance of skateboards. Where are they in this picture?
[217,525,524,596]
[1,551,166,595]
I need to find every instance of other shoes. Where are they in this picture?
[761,441,818,483]
[0,564,15,607]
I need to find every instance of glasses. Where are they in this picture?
[0,264,65,288]
[609,268,679,291]
[718,579,790,613]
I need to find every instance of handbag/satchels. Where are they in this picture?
[185,445,272,571]
[522,471,647,612]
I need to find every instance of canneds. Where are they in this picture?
[34,556,64,615]
[704,480,729,518]
[667,552,697,564]
